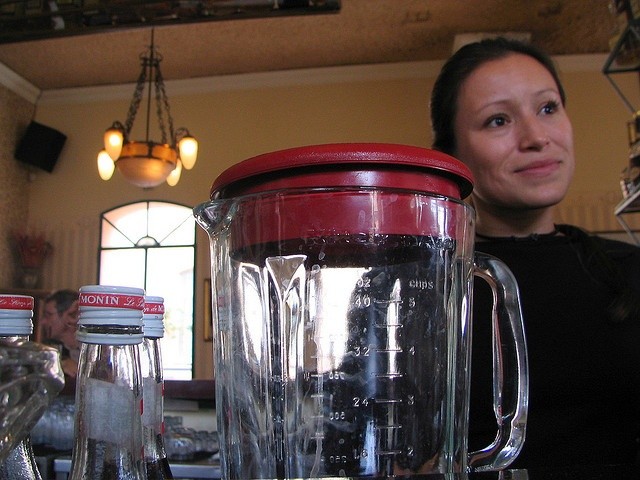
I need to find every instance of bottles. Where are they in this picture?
[67,285,149,480]
[0,295,42,480]
[144,296,175,480]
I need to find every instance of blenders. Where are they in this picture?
[193,142,530,480]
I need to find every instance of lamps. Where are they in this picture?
[96,26,199,189]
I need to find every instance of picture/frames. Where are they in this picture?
[203,277,215,341]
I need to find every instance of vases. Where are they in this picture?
[21,267,40,288]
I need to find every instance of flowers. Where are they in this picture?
[12,232,52,267]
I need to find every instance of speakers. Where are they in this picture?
[13,121,68,173]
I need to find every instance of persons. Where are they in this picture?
[42,289,83,392]
[346,39,639,480]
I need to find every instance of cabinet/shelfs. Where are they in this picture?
[600,21,640,245]
